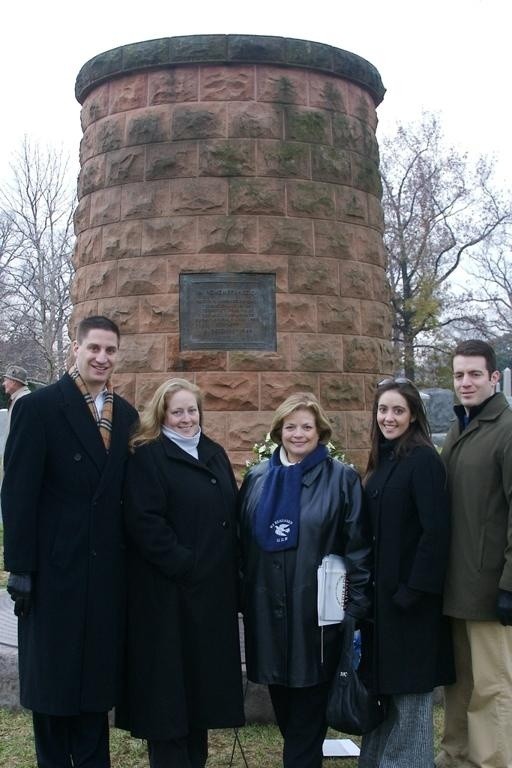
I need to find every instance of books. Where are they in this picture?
[316,554,349,626]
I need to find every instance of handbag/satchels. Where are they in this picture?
[325,603,387,735]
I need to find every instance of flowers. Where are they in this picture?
[241,429,356,480]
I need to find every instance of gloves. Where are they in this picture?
[498,593,512,625]
[6,572,32,612]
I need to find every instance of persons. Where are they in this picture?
[233,392,373,766]
[350,378,460,767]
[115,380,251,767]
[3,314,142,767]
[2,366,34,420]
[433,336,512,768]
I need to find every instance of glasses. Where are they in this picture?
[376,378,412,388]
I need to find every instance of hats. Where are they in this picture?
[3,365,32,385]
